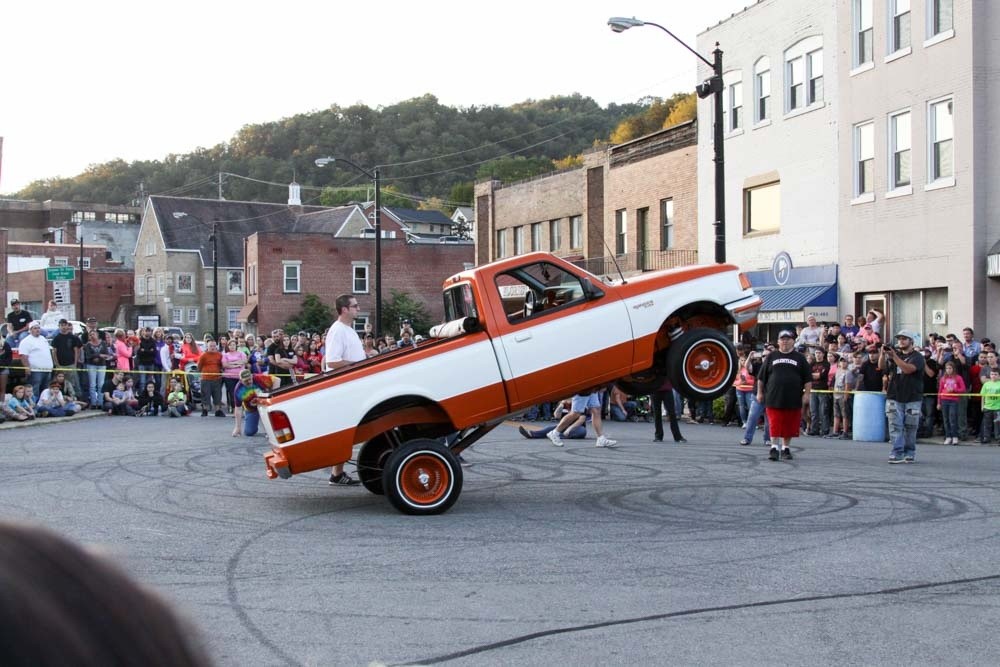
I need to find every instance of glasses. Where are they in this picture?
[344,302,360,308]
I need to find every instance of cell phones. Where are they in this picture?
[944,348,954,352]
[753,352,762,357]
[883,346,891,351]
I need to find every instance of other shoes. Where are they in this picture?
[651,438,687,443]
[806,431,849,439]
[943,437,958,445]
[974,437,991,443]
[201,410,227,417]
[518,425,533,438]
[740,439,772,445]
[888,457,915,463]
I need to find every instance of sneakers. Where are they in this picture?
[455,456,473,467]
[546,431,564,447]
[596,438,617,447]
[768,447,792,460]
[328,471,362,486]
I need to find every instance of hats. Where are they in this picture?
[239,369,252,382]
[864,324,872,329]
[58,319,68,324]
[86,317,97,322]
[894,329,914,340]
[929,332,938,337]
[50,381,61,387]
[28,321,40,329]
[10,299,21,305]
[869,346,879,353]
[778,330,794,338]
[839,356,848,362]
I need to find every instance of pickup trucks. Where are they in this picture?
[249,251,762,517]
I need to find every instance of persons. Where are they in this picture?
[867,307,885,332]
[0,299,423,421]
[324,294,370,485]
[232,369,281,441]
[878,328,924,465]
[506,309,525,320]
[519,375,686,447]
[757,330,811,461]
[0,516,210,667]
[688,314,1000,445]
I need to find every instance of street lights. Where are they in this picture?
[47,226,84,322]
[172,211,219,345]
[314,156,382,346]
[608,15,729,261]
